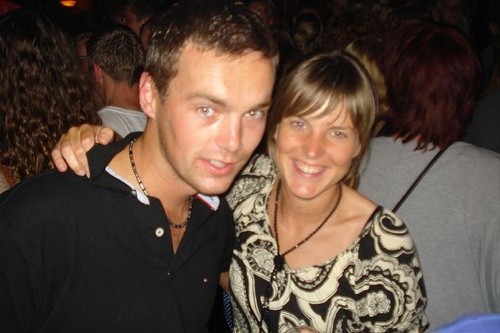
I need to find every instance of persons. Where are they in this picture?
[0,0,500,333]
